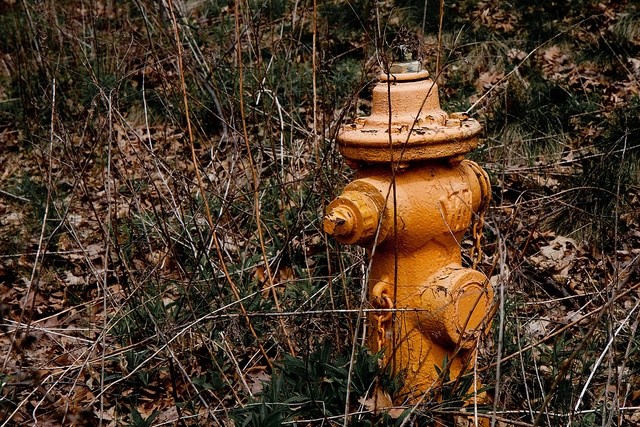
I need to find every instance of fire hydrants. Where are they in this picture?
[320,58,498,427]
